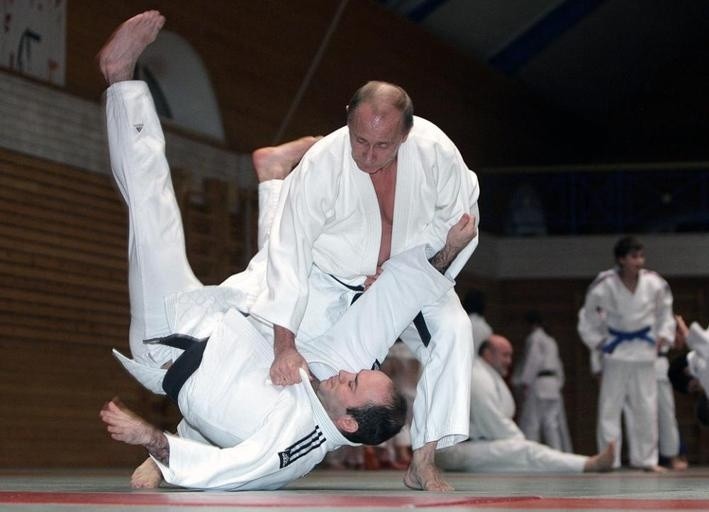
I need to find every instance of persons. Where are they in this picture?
[93,9,480,490]
[319,339,419,469]
[128,79,481,492]
[434,334,617,474]
[457,285,493,346]
